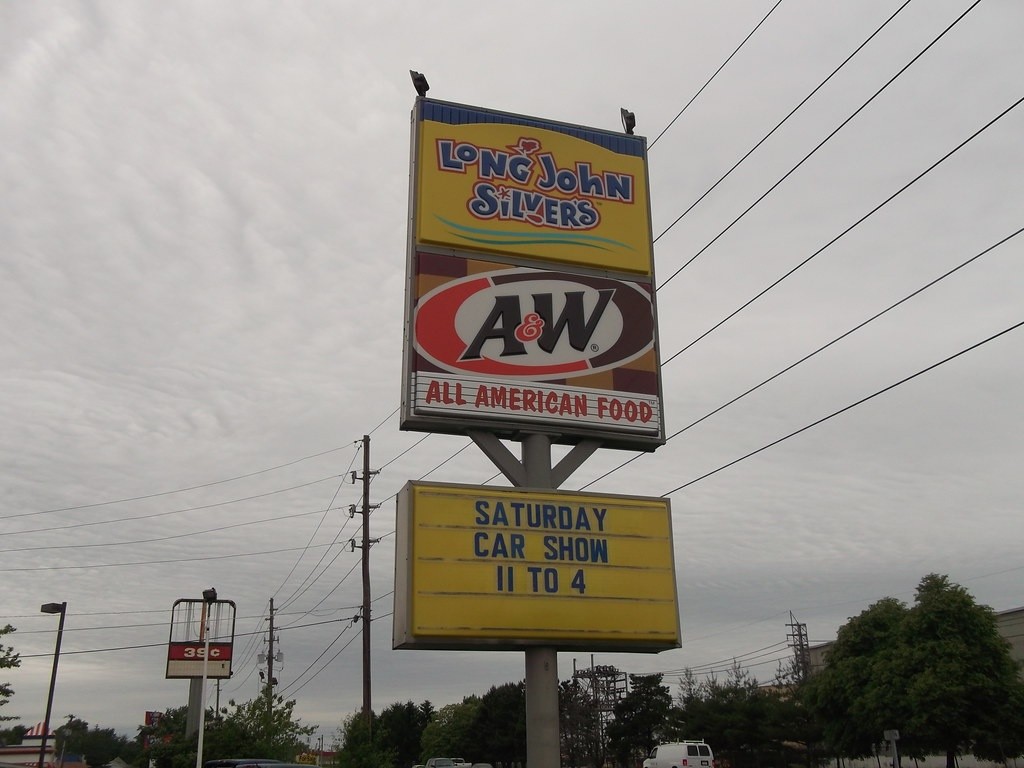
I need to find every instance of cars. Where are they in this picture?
[203,758,322,768]
[412,757,493,768]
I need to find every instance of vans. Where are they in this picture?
[642,738,715,768]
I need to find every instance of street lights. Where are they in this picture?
[39,600,68,768]
